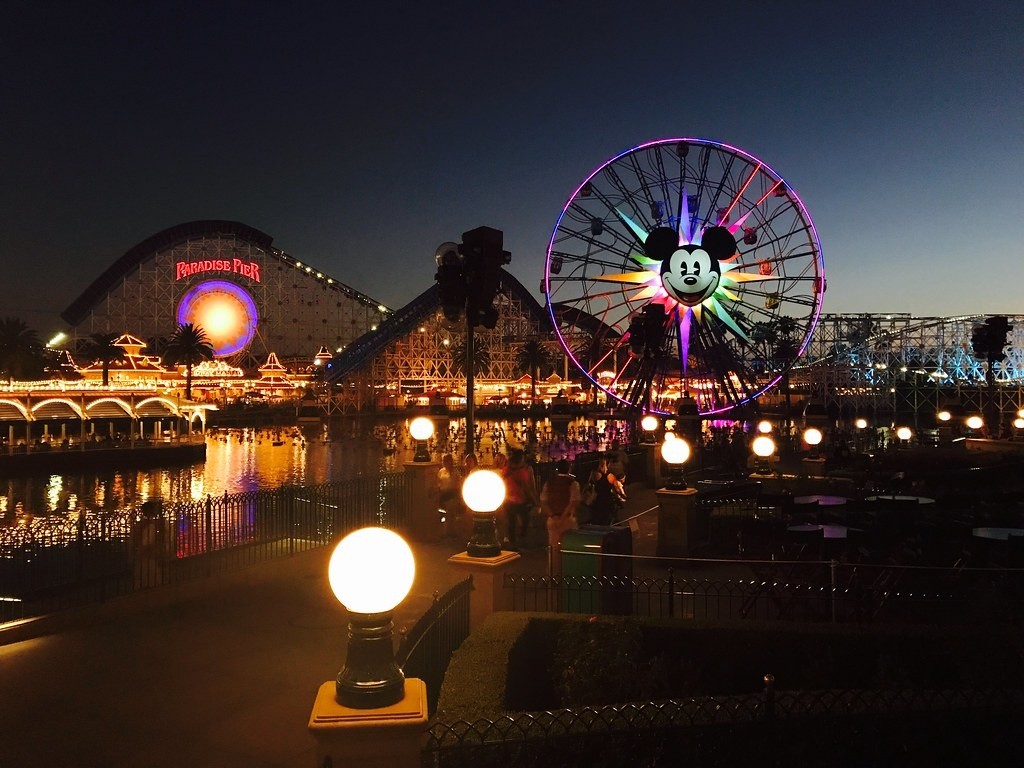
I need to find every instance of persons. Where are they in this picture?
[436,448,628,575]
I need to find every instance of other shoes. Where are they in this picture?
[508,535,516,543]
[521,532,528,537]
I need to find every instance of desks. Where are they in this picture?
[786,525,864,618]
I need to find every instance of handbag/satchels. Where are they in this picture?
[582,482,595,506]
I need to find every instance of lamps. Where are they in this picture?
[805,428,823,459]
[752,437,775,474]
[462,471,506,558]
[967,417,983,439]
[939,412,950,427]
[642,417,658,444]
[409,417,433,462]
[659,441,690,490]
[326,527,416,709]
[759,421,772,438]
[897,428,912,448]
[856,419,866,433]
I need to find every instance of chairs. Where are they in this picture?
[737,530,809,617]
